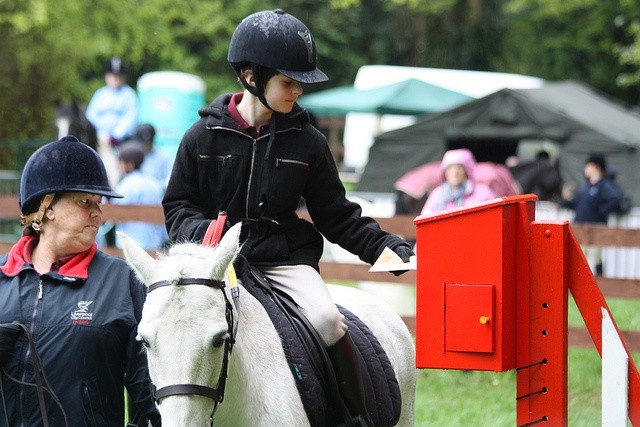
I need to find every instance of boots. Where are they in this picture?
[326,330,374,426]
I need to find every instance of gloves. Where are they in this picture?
[193,219,230,244]
[388,245,416,277]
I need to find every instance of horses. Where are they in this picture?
[114,222,417,427]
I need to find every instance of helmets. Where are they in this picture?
[19,135,124,232]
[227,9,329,113]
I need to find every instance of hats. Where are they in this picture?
[586,151,606,177]
[137,124,156,142]
[117,141,145,166]
[105,55,126,77]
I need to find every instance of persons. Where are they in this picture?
[1,135,162,427]
[102,123,170,250]
[557,154,632,225]
[162,8,415,426]
[85,57,138,145]
[419,149,500,216]
